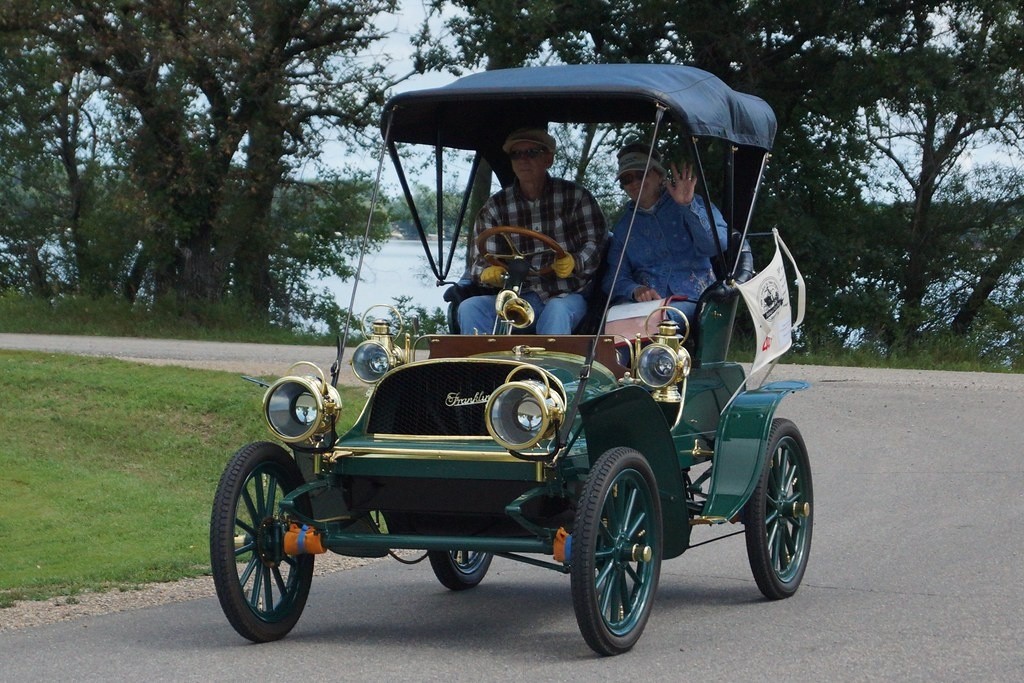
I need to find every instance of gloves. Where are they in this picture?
[551,251,574,279]
[481,266,507,288]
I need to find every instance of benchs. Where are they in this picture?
[443,231,753,372]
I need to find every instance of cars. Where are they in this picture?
[208,61,815,661]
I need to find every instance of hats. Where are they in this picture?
[503,128,556,154]
[614,143,667,181]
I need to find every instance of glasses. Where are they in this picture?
[508,148,543,160]
[621,171,648,184]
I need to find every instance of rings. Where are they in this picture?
[687,176,693,180]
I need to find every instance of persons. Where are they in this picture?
[602,143,728,369]
[458,130,609,337]
